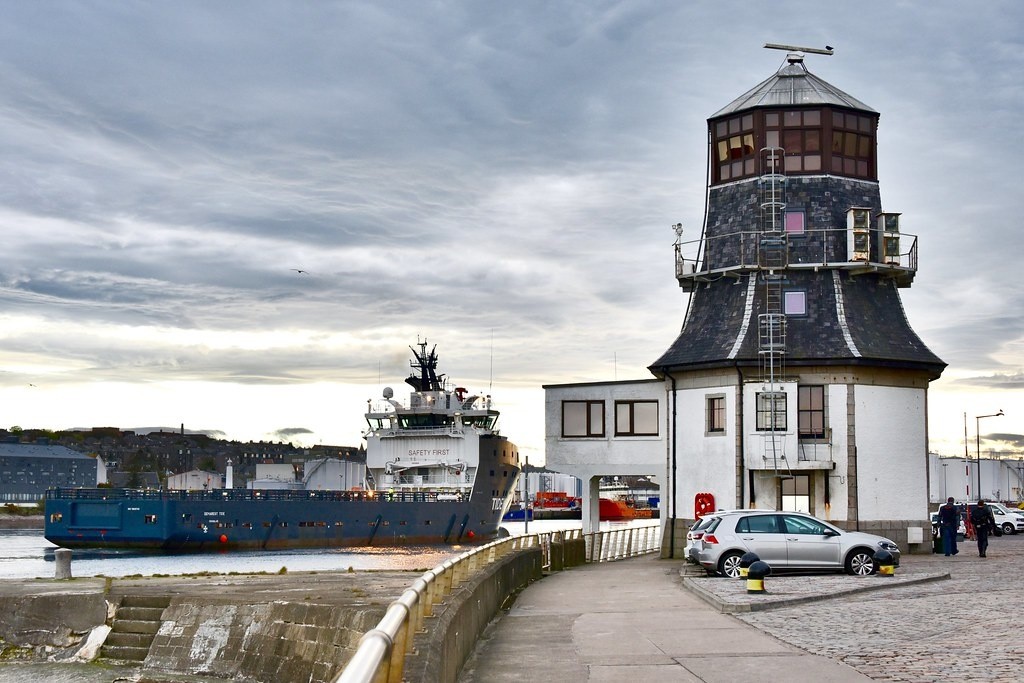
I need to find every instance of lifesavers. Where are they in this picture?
[696,498,710,515]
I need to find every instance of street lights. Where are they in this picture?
[976,413,1005,500]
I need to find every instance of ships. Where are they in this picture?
[44,335,524,555]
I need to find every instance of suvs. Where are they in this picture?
[689,510,900,580]
[930,503,1024,540]
[684,509,827,566]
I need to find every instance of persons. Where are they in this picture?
[970,500,994,557]
[937,497,960,556]
